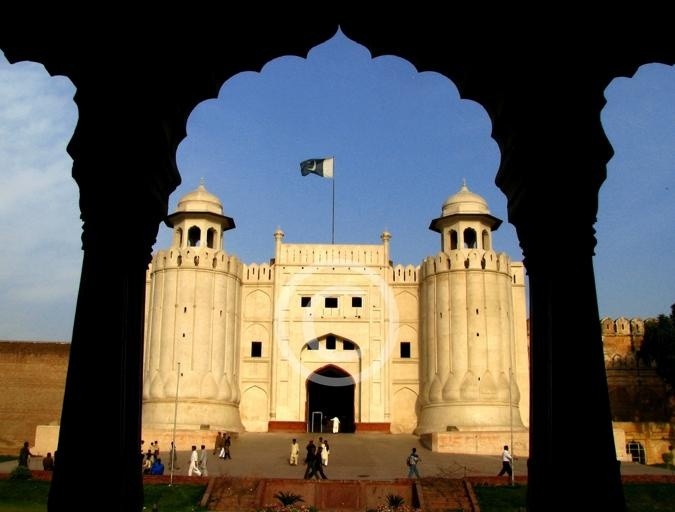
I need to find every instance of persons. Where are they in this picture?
[330,416,341,433]
[497,445,514,475]
[18,441,42,469]
[289,438,301,466]
[407,447,422,478]
[303,436,331,479]
[141,430,233,477]
[43,452,54,470]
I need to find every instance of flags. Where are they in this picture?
[299,157,333,178]
